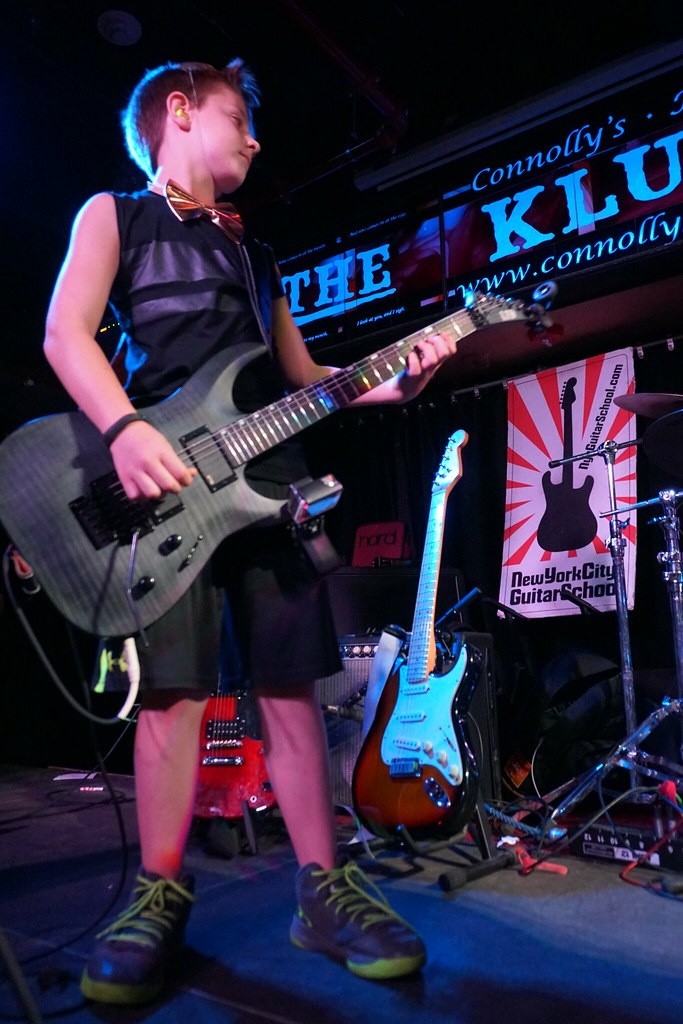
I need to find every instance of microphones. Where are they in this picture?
[321,704,364,723]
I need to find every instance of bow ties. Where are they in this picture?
[164,178,243,245]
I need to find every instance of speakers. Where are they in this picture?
[260,631,503,829]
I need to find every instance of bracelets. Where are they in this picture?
[102,412,152,449]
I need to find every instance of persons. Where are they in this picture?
[42,57,458,1003]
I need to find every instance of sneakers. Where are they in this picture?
[288,858,427,976]
[80,866,196,1002]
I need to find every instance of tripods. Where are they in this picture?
[498,438,683,843]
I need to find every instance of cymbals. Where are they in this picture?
[612,392,683,420]
[641,409,683,479]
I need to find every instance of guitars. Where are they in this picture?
[350,428,487,845]
[193,587,278,821]
[0,294,548,640]
